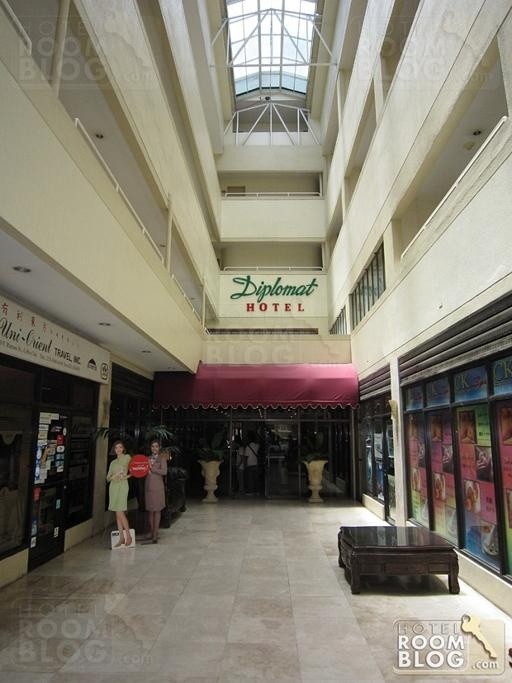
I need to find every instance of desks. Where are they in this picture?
[337,523,463,600]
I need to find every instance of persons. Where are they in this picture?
[143,440,168,544]
[234,434,261,497]
[105,441,133,548]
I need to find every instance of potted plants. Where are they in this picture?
[298,430,331,503]
[196,430,227,502]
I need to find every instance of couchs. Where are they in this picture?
[135,475,188,530]
